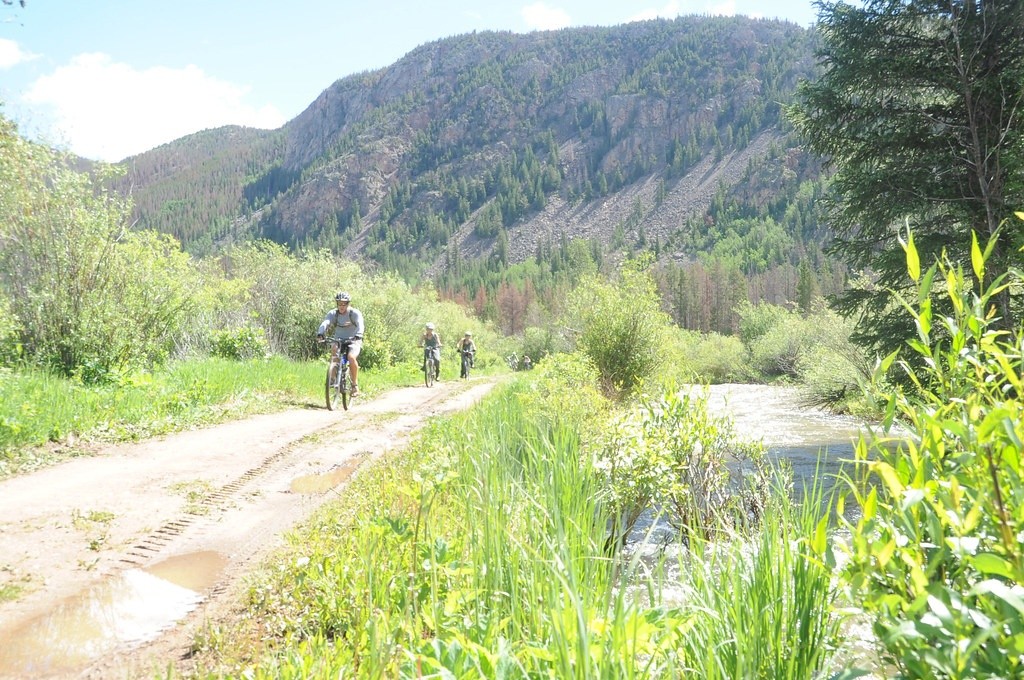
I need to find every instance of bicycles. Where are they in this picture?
[317,337,355,412]
[524,362,528,371]
[459,350,473,380]
[512,361,518,372]
[418,346,440,388]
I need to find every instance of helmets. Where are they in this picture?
[425,322,435,330]
[335,291,351,302]
[464,330,472,337]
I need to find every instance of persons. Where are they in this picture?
[420,322,442,381]
[457,332,477,378]
[510,352,519,369]
[524,356,531,369]
[314,292,364,397]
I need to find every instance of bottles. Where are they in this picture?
[342,352,346,371]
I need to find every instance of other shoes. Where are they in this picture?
[421,366,425,370]
[436,375,440,381]
[472,364,474,368]
[351,385,359,397]
[326,378,335,386]
[461,373,466,379]
